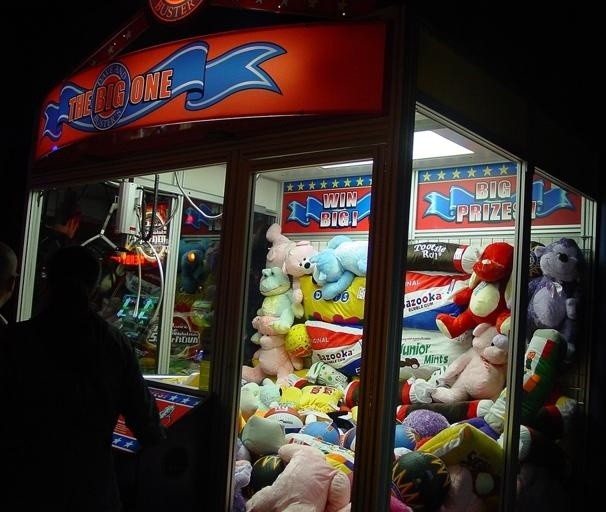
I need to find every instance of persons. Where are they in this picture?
[0,242,17,308]
[37,199,82,269]
[0,245,167,512]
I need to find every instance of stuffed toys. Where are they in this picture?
[229,221,586,512]
[231,221,587,511]
